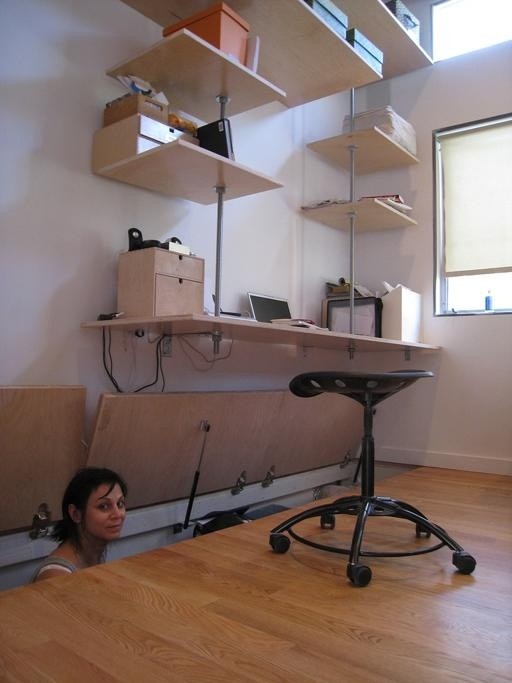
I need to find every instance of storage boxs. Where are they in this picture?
[384,1,421,46]
[161,2,251,67]
[304,1,350,40]
[103,92,170,126]
[346,29,385,77]
[93,113,200,172]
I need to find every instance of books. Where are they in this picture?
[271,318,329,331]
[197,119,234,161]
[246,36,260,73]
[326,277,375,297]
[344,105,417,156]
[358,195,413,214]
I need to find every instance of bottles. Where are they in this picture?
[484,291,493,310]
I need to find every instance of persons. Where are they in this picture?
[30,467,127,582]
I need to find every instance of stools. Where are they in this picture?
[265,370,477,587]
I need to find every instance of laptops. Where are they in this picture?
[248,292,292,323]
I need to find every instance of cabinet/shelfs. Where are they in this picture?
[115,248,205,320]
[82,0,443,352]
